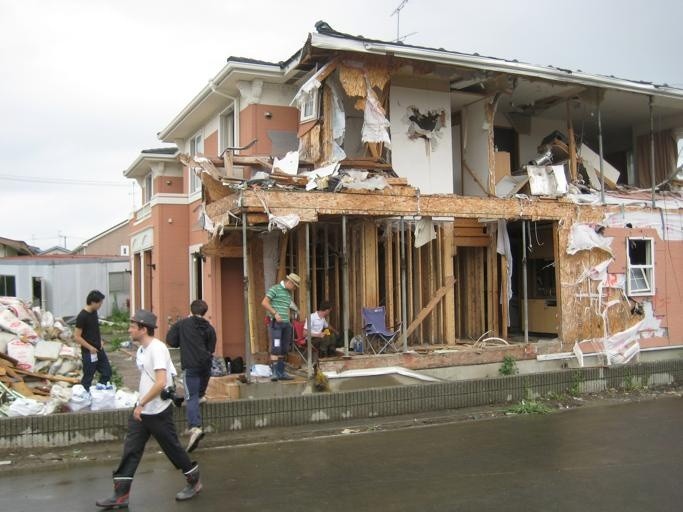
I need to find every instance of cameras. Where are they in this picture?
[160,386,184,408]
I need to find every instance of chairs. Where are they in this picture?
[291,319,318,365]
[360,306,403,356]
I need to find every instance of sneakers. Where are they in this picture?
[319,349,341,358]
[186,429,205,452]
[199,396,207,404]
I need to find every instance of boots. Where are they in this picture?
[176,465,204,501]
[271,360,295,381]
[96,477,134,508]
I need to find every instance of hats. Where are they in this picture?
[286,273,301,287]
[129,310,158,328]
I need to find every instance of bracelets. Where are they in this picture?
[271,310,277,316]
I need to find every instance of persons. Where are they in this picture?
[166,300,216,453]
[74,290,112,391]
[303,301,339,367]
[261,272,301,381]
[95,309,203,509]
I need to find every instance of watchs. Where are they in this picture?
[135,401,144,408]
